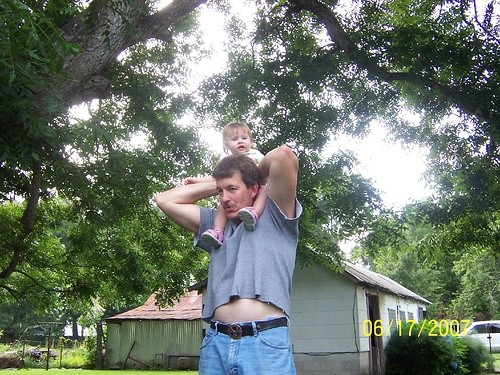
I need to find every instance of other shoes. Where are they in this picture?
[201,229,224,249]
[237,206,258,231]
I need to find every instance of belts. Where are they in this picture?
[210,317,286,338]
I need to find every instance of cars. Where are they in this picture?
[458,319,500,354]
[22,347,58,360]
[23,325,50,338]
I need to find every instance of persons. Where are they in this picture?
[155,145,303,375]
[184,123,269,250]
